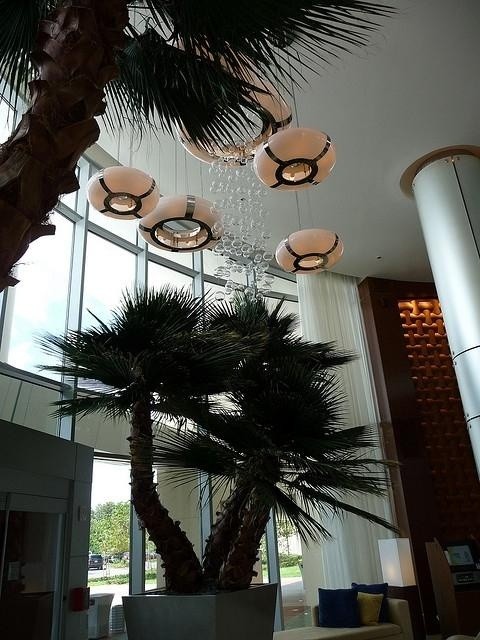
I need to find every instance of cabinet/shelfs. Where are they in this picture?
[426,534,480,640]
[0,591,53,640]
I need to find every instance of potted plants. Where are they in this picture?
[35,282,402,640]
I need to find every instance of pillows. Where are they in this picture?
[357,592,384,626]
[318,588,361,628]
[352,583,388,623]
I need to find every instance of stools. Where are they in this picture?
[108,605,125,634]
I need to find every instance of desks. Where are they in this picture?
[88,593,115,640]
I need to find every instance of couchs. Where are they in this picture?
[273,597,414,640]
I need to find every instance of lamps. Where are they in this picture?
[87,166,160,221]
[377,538,426,640]
[254,127,336,191]
[174,78,293,167]
[276,228,345,275]
[138,194,224,253]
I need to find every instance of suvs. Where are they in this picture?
[88,554,103,569]
